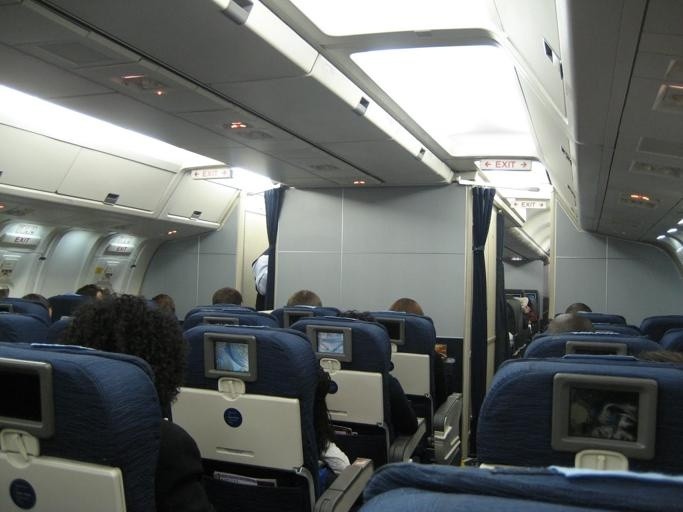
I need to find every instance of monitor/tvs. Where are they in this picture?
[550,372,658,461]
[204,332,257,383]
[0,358,56,439]
[283,310,314,328]
[566,340,629,357]
[307,325,352,363]
[375,317,406,346]
[203,316,239,326]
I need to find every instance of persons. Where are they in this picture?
[497,292,683,365]
[251,248,271,310]
[0,281,451,512]
